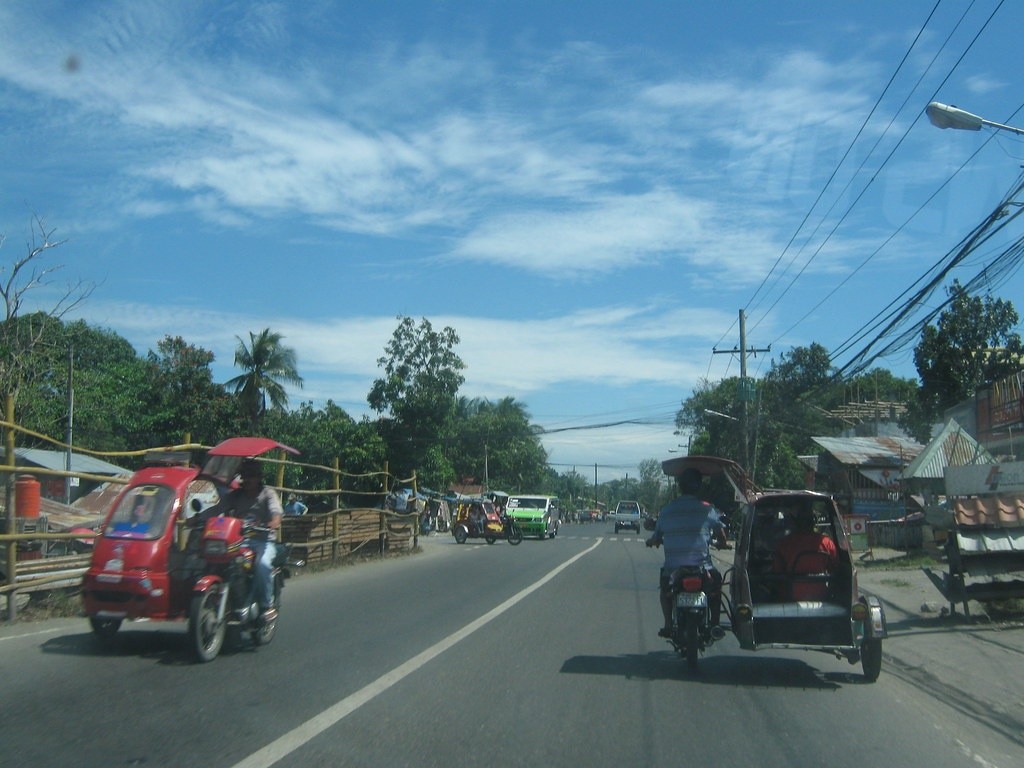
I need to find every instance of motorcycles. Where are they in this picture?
[77,437,305,660]
[643,456,887,685]
[451,499,523,544]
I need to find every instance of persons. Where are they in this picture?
[643,468,729,634]
[284,493,308,516]
[772,506,837,603]
[111,493,156,534]
[174,460,284,623]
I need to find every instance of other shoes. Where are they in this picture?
[657,629,670,637]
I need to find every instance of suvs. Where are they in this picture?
[580,510,602,522]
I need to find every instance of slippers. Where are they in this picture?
[263,607,277,621]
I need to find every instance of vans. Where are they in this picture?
[506,495,559,540]
[614,501,642,533]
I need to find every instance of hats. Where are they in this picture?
[288,494,296,500]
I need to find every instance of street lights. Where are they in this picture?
[702,408,752,488]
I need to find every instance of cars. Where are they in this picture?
[603,511,616,520]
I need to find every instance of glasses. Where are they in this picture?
[240,471,259,478]
